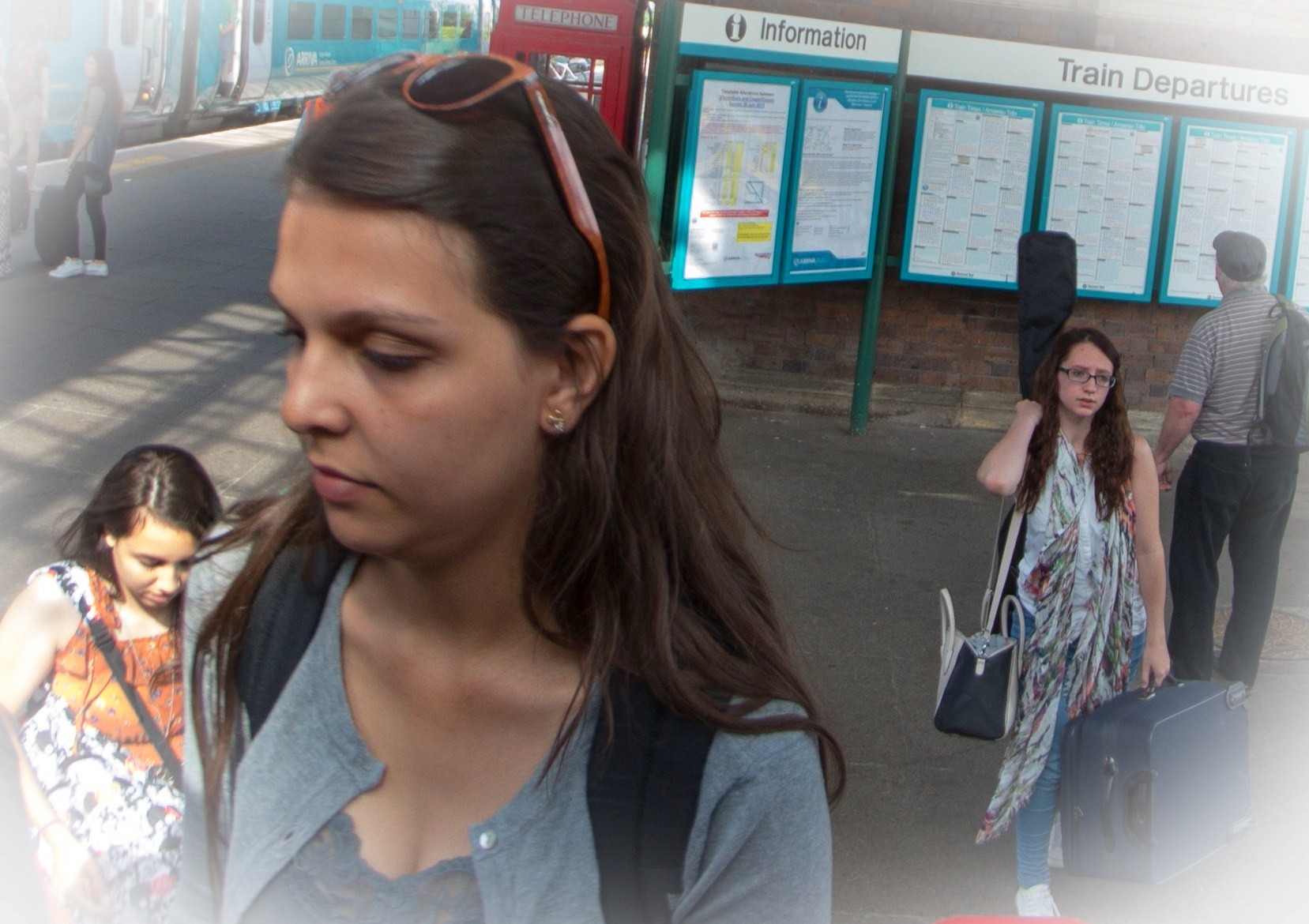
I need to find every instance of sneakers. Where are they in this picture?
[82,260,108,277]
[49,256,84,278]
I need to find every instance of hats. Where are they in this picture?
[1213,230,1266,281]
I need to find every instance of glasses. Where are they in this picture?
[1057,367,1116,388]
[322,51,611,321]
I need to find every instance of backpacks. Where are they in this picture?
[1246,293,1309,453]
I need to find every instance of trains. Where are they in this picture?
[4,0,500,160]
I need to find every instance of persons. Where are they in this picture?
[183,54,849,924]
[976,329,1171,916]
[48,49,123,279]
[0,447,227,924]
[1151,231,1309,699]
[5,24,49,191]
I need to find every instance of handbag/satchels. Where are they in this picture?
[932,632,1025,742]
[84,161,113,198]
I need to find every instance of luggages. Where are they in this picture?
[10,170,30,231]
[35,185,69,267]
[1058,672,1256,881]
